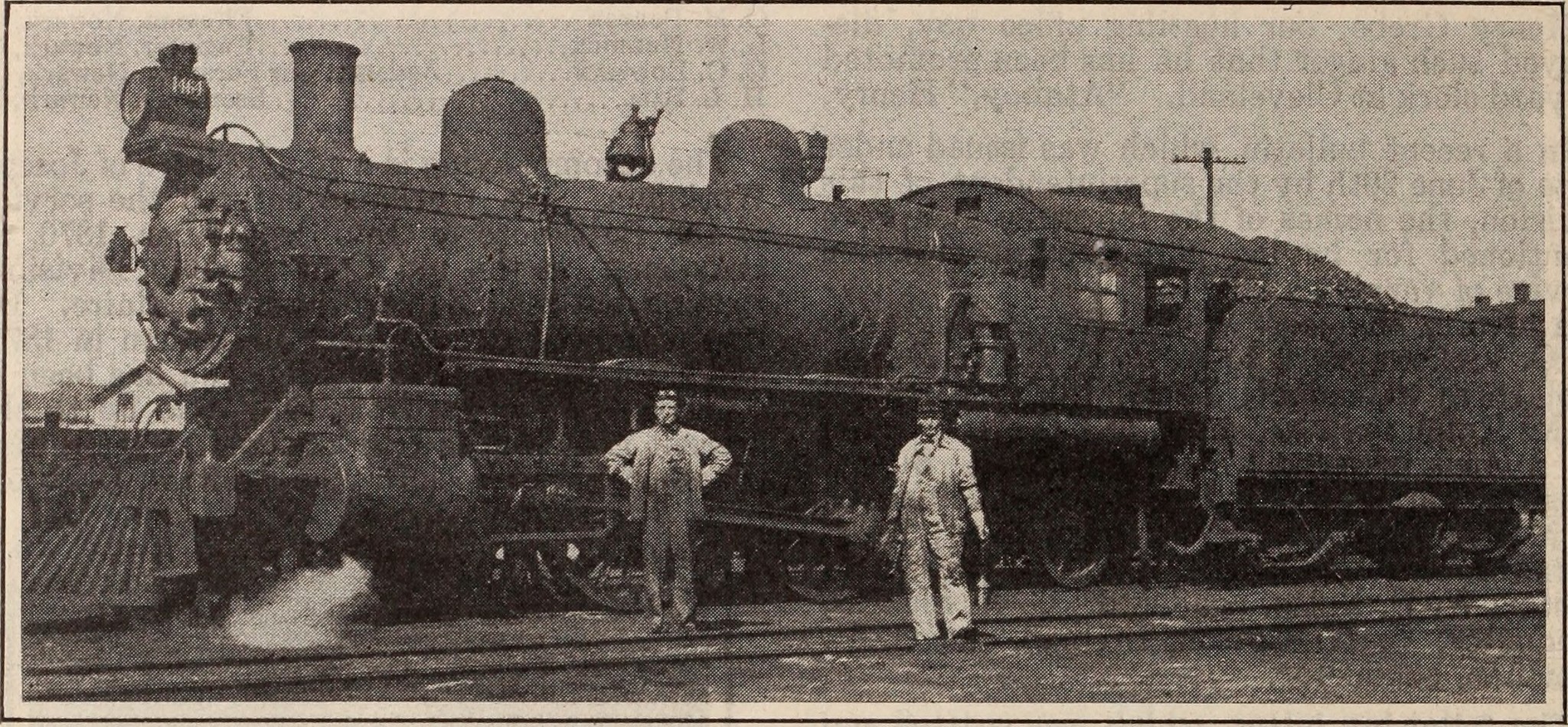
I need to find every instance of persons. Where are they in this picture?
[602,389,733,633]
[887,404,989,640]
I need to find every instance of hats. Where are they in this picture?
[655,388,679,399]
[916,397,944,416]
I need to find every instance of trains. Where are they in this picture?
[62,35,1547,627]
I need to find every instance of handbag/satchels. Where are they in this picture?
[876,523,903,571]
